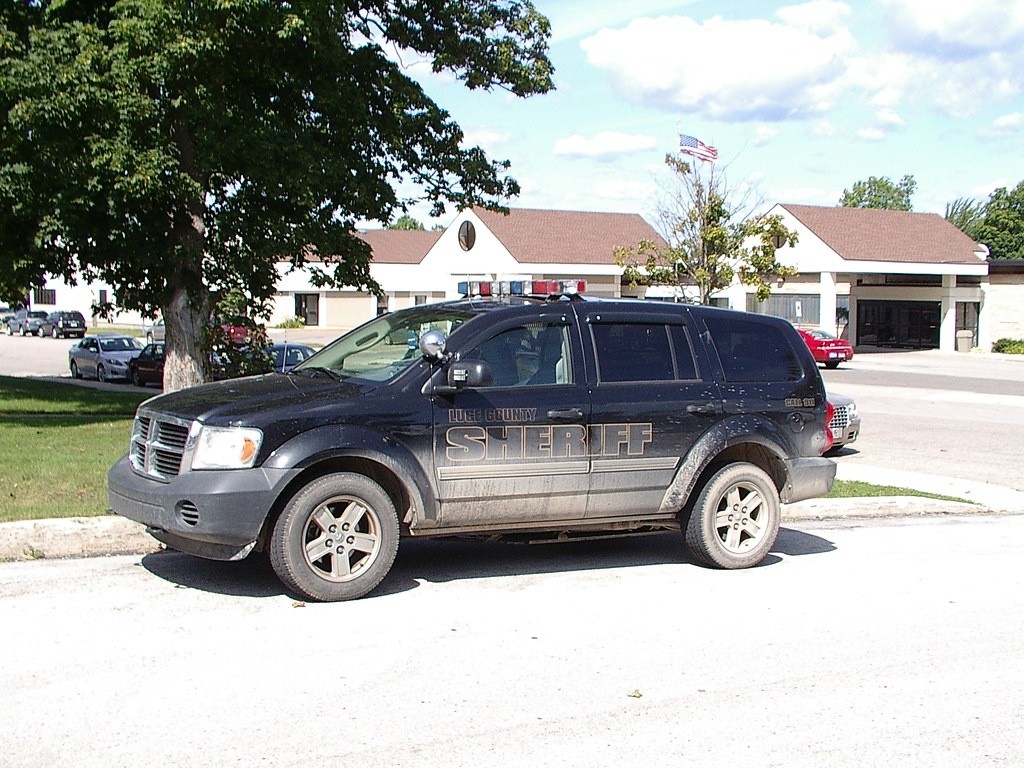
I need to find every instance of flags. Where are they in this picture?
[680,134,717,163]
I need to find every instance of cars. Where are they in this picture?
[147,315,266,346]
[384,325,447,345]
[230,344,318,374]
[129,343,230,387]
[826,392,861,451]
[68,335,150,383]
[796,328,854,368]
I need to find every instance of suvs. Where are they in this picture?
[108,278,837,602]
[0,307,87,339]
